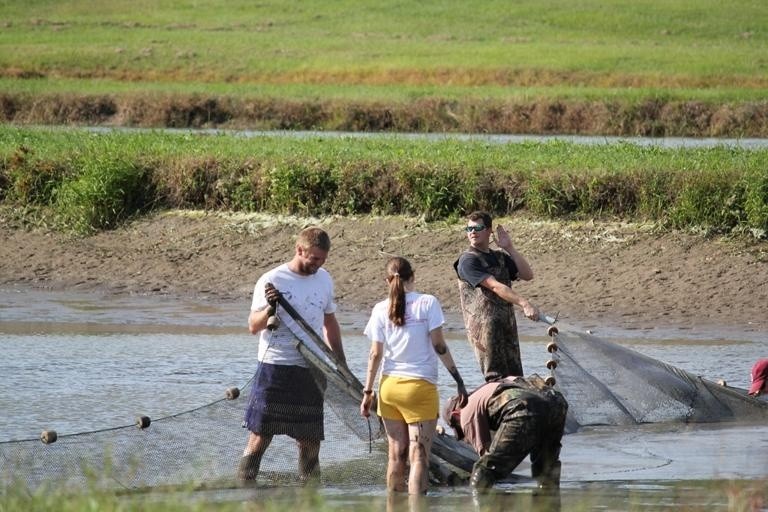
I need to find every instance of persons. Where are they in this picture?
[357,256,469,495]
[440,373,566,493]
[746,356,768,398]
[451,210,540,376]
[236,225,350,486]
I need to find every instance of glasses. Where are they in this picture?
[465,226,487,232]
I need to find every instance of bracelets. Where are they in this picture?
[363,388,373,395]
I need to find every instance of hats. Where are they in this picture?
[747,359,768,395]
[442,395,465,441]
